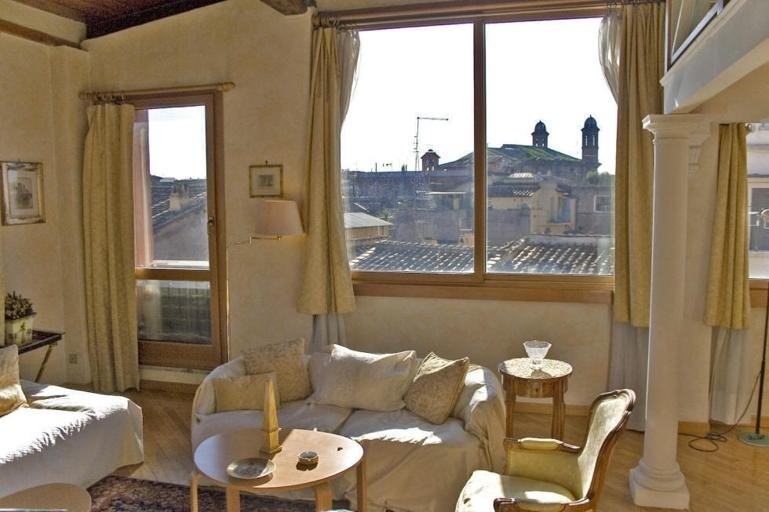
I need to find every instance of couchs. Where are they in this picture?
[191,355,508,512]
[0,378,146,500]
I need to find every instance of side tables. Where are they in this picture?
[499,356,574,443]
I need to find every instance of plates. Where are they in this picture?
[226,457,276,480]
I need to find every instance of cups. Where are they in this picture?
[523,341,552,370]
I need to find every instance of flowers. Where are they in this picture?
[5,290,34,320]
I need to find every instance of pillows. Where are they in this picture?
[239,336,312,403]
[306,341,419,412]
[212,371,281,413]
[0,344,27,419]
[403,351,470,426]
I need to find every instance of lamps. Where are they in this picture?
[233,200,307,245]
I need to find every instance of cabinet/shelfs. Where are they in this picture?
[1,329,62,383]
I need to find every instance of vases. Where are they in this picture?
[4,312,37,348]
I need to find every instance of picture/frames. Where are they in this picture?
[1,160,47,225]
[249,164,284,198]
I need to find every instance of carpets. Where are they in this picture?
[89,476,351,511]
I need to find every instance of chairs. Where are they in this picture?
[0,482,93,511]
[454,387,637,512]
